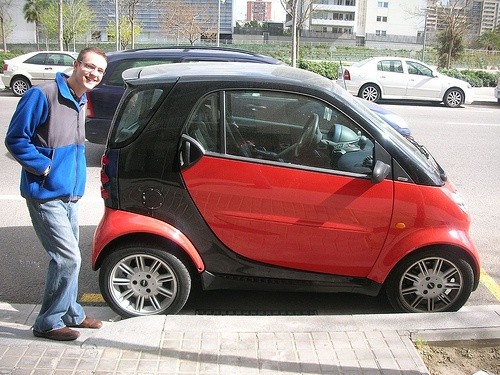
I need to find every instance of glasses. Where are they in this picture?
[78,60,106,76]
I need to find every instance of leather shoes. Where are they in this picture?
[33,326,80,341]
[66,317,102,328]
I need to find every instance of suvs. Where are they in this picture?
[87,46,410,145]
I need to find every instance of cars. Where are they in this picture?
[92,62,482,316]
[494,77,500,106]
[337,56,475,108]
[2,51,81,97]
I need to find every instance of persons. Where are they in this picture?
[4,47,108,340]
